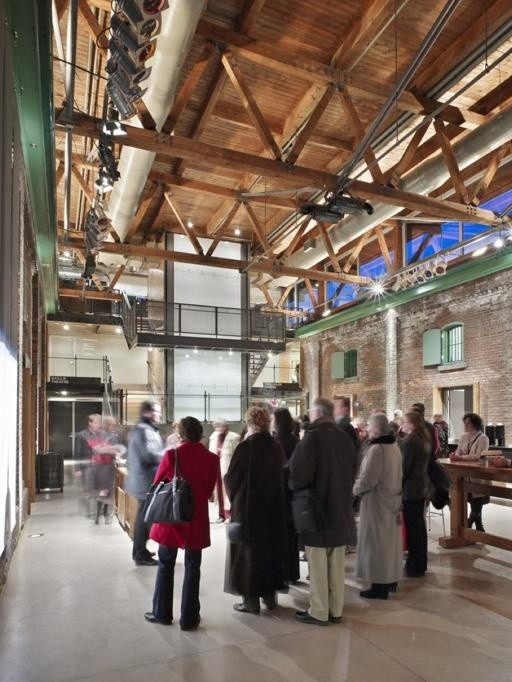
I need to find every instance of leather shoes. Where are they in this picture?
[294,611,343,626]
[233,603,248,612]
[360,586,396,599]
[133,552,157,566]
[144,612,171,625]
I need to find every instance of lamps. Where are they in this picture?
[83,0,169,258]
[300,0,461,224]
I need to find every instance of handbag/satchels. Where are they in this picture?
[228,521,250,546]
[430,462,451,493]
[290,496,320,534]
[140,478,194,524]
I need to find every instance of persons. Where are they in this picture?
[77,400,242,566]
[224,396,491,626]
[144,416,219,631]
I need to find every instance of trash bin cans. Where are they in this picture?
[37,453,64,494]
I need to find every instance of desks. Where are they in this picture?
[435,457,512,551]
